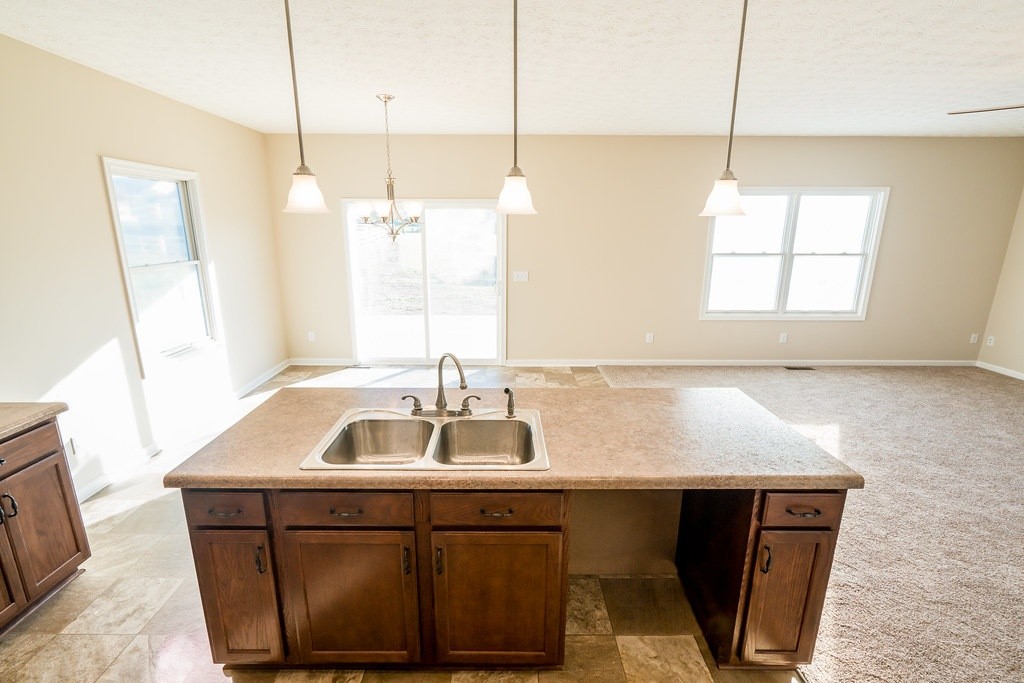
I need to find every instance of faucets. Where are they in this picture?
[433,353,467,408]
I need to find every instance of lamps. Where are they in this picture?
[698,0,748,216]
[496,0,537,215]
[282,0,330,213]
[358,93,421,242]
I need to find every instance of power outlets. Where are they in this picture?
[987,336,994,347]
[647,333,654,343]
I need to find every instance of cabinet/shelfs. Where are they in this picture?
[0,416,92,639]
[182,488,573,671]
[675,489,847,670]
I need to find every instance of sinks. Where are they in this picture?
[299,407,435,468]
[433,408,551,472]
